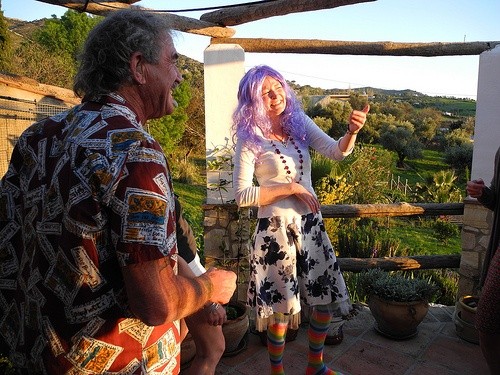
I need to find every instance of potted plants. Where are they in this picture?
[220,302,249,352]
[325,302,365,345]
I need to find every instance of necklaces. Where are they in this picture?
[270,129,303,183]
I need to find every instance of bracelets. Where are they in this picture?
[204,302,219,313]
[347,123,358,135]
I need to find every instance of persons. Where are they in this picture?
[232,65,370,375]
[1,6,237,375]
[173,193,229,374]
[465,146,500,375]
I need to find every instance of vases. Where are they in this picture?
[358,266,437,340]
[452,295,481,346]
